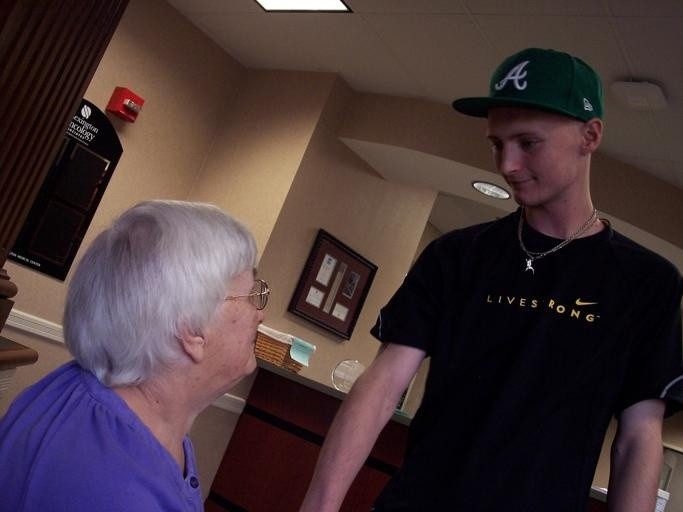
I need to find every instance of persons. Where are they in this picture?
[292,46,682,512]
[0,197,270,511]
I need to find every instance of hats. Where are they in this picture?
[450,47,606,124]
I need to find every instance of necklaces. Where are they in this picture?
[518,205,598,276]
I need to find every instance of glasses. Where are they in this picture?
[224,278,271,311]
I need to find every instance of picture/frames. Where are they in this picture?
[286,229,379,341]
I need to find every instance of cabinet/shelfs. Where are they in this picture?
[205,357,412,511]
